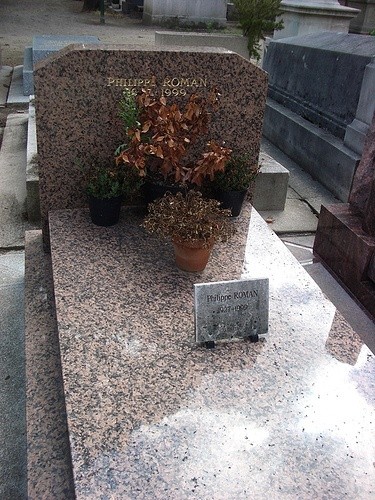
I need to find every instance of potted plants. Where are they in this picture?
[196,148,264,217]
[68,150,133,227]
[112,74,234,217]
[139,187,233,272]
